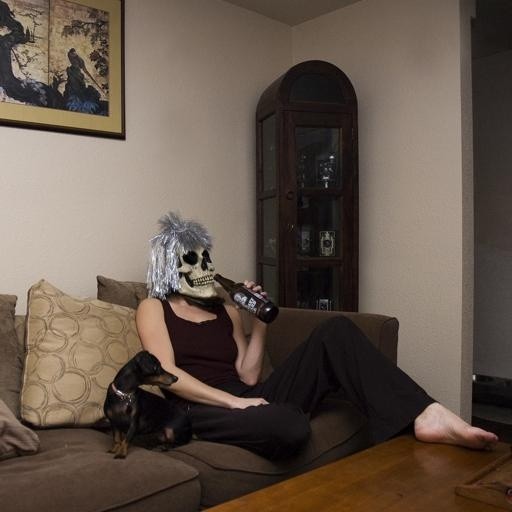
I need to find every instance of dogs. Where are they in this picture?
[102,350,199,460]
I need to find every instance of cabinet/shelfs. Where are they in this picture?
[256,60,359,311]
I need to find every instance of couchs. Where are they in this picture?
[1,307,401,512]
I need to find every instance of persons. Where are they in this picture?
[136,211,499,465]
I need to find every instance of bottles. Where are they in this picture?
[213,273,279,323]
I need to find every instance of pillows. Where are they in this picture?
[0,275,155,464]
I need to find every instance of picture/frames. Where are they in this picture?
[0,1,125,141]
